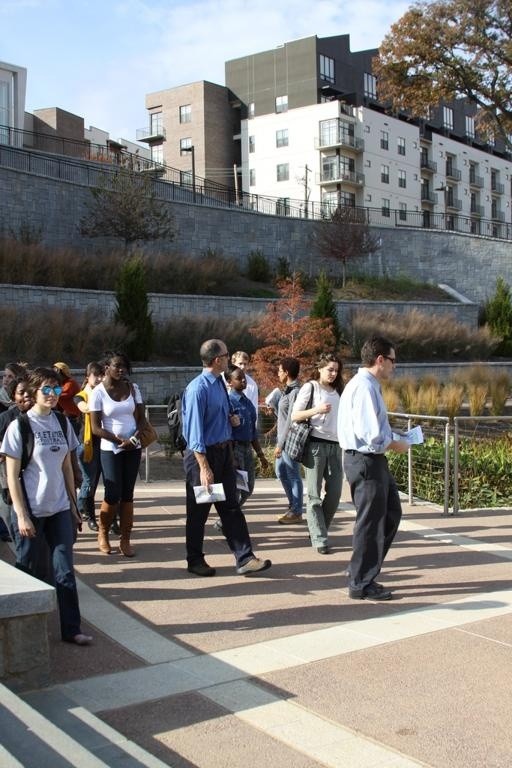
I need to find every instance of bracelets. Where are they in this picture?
[256,452,264,458]
[129,436,139,447]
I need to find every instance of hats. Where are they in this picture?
[53,362,72,378]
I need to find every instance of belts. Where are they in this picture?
[345,449,358,455]
[239,440,252,447]
[211,441,233,450]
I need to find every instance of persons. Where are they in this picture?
[51,363,82,436]
[0,366,94,645]
[76,360,121,535]
[290,354,343,556]
[267,358,305,525]
[214,365,269,535]
[336,338,411,602]
[0,362,31,412]
[0,376,36,577]
[181,339,271,575]
[90,353,148,556]
[220,350,258,430]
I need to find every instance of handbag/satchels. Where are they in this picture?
[284,421,314,463]
[133,403,159,448]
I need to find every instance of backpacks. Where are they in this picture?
[167,390,187,457]
[0,411,68,505]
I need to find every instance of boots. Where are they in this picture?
[80,497,99,531]
[111,513,121,536]
[119,502,136,557]
[97,499,119,554]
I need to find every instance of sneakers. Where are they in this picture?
[187,556,216,576]
[236,560,272,575]
[279,504,303,524]
[213,519,222,534]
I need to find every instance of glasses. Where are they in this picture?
[36,386,63,397]
[377,353,395,363]
[213,352,230,360]
[278,370,284,376]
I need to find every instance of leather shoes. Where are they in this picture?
[318,547,329,554]
[348,584,392,600]
[349,591,392,600]
[63,633,93,644]
[344,571,382,588]
